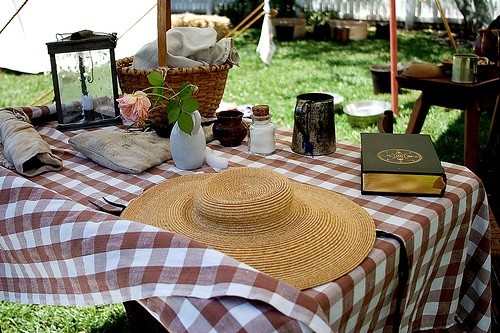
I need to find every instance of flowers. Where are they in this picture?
[116,73,207,133]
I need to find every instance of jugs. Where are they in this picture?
[478,28,500,69]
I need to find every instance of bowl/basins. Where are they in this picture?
[311,91,344,110]
[343,100,391,128]
[401,61,444,79]
[440,59,495,75]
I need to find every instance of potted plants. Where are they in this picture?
[312,10,341,40]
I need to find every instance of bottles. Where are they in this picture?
[246,104,277,155]
[212,109,247,147]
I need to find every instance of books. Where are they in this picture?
[361,133,446,198]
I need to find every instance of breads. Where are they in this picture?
[402,62,440,78]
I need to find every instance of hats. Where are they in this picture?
[120,167,377,290]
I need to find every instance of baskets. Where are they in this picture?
[115,55,234,118]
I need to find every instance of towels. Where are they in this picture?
[256,0,276,67]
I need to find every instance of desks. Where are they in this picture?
[0,100,500,333]
[395,62,500,181]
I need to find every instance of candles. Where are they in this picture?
[81,94,94,109]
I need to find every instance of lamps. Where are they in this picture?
[171,108,206,169]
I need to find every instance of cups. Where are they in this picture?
[291,92,336,156]
[451,53,490,84]
